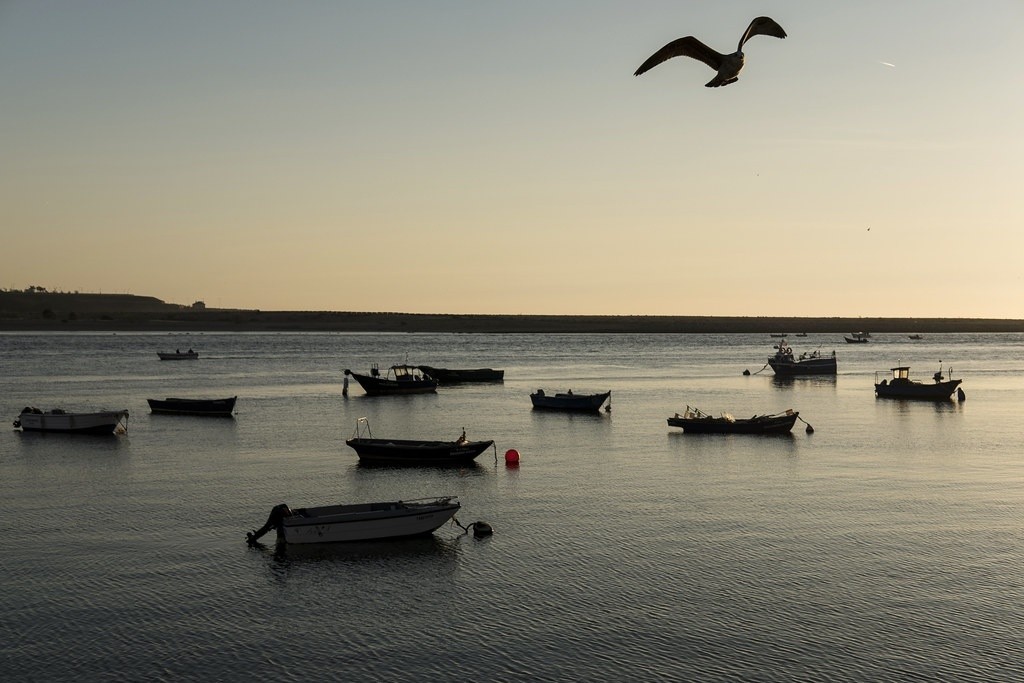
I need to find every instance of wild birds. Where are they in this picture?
[633,17,787,88]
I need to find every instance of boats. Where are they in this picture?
[843,336,870,343]
[146,395,238,419]
[908,334,923,339]
[873,358,964,403]
[345,417,497,465]
[341,363,440,398]
[418,365,506,386]
[795,332,808,337]
[771,342,837,376]
[157,348,199,361]
[770,333,788,337]
[246,495,460,551]
[667,404,801,437]
[850,330,872,338]
[529,388,613,416]
[13,406,132,435]
